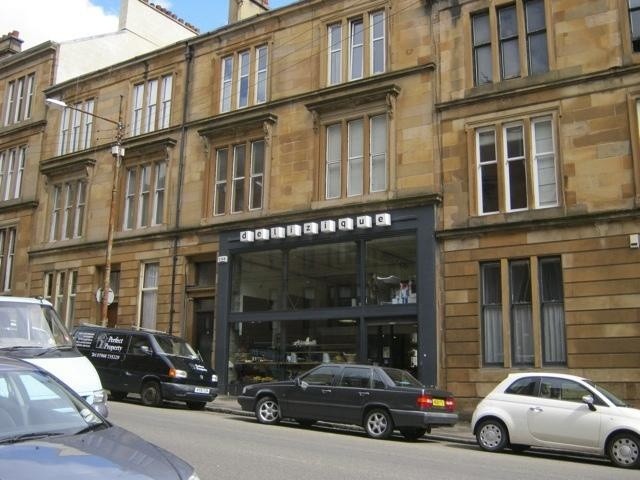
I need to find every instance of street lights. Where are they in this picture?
[45,96,124,328]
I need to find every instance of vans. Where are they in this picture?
[72,326,222,410]
[0,292,109,421]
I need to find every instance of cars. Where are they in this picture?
[0,355,203,480]
[472,370,640,470]
[235,363,458,441]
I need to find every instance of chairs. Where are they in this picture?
[0,395,26,433]
[130,341,150,353]
